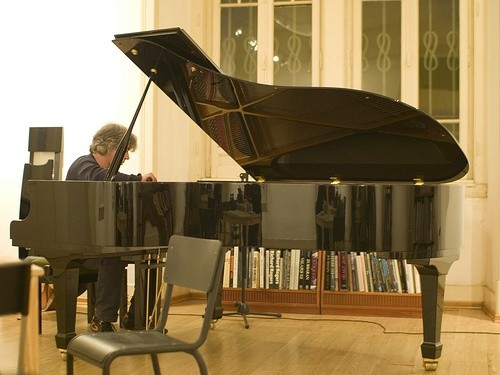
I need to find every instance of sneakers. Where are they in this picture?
[121,312,169,335]
[91,315,117,332]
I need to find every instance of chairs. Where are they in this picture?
[66,234,226,375]
[0,126,127,375]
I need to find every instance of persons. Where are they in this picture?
[110,182,173,247]
[314,184,346,247]
[64,124,168,333]
[185,183,262,246]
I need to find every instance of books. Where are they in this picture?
[324,250,421,294]
[246,246,318,290]
[221,245,242,288]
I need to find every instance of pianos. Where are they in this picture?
[9,27,469,372]
